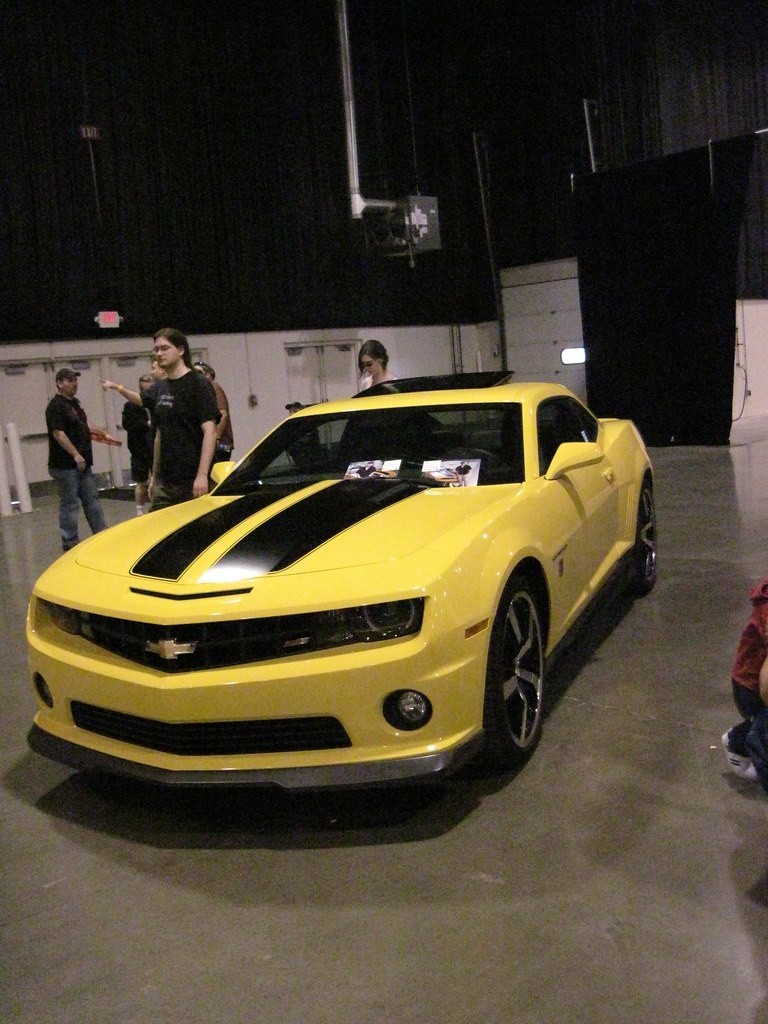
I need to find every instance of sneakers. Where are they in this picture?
[722,728,757,780]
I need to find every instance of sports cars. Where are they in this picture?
[28,369,660,797]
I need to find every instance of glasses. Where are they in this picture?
[195,362,205,365]
[361,358,379,368]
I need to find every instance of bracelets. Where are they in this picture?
[118,385,122,393]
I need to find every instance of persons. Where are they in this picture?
[45,368,118,552]
[722,577,768,786]
[456,462,471,487]
[355,461,375,477]
[97,327,398,519]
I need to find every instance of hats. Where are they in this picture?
[56,368,81,382]
[286,402,303,410]
